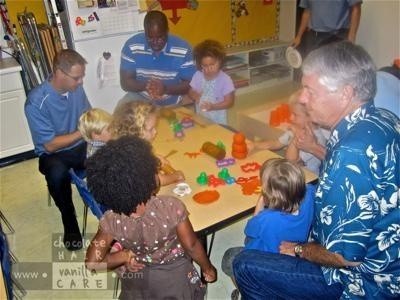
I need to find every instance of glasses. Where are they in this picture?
[62,70,86,81]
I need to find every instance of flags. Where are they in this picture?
[160,104,291,204]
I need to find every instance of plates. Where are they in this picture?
[286,47,302,69]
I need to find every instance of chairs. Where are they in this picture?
[1,163,121,299]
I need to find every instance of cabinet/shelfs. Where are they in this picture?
[222,39,295,96]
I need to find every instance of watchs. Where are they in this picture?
[293,242,303,257]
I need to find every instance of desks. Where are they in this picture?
[152,106,319,289]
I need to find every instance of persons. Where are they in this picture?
[288,0,364,82]
[233,42,400,299]
[79,107,110,158]
[109,98,185,187]
[113,11,196,116]
[24,49,92,252]
[243,89,326,174]
[96,52,113,89]
[83,134,218,300]
[222,156,314,300]
[188,40,235,128]
